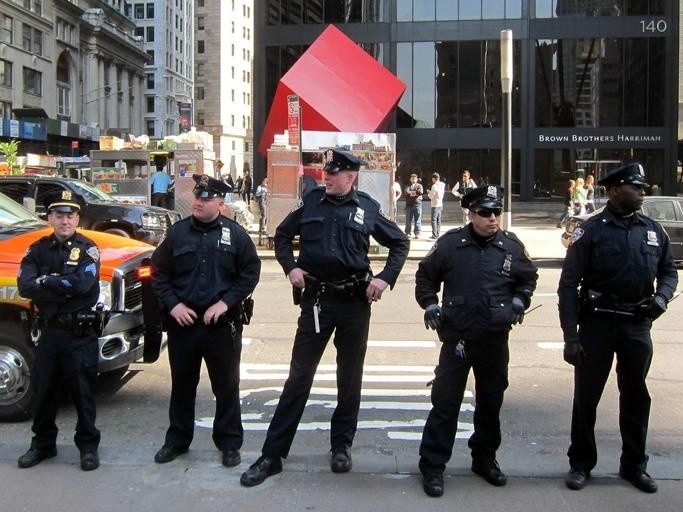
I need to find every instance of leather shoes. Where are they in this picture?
[154,444,190,464]
[565,466,592,490]
[80,450,100,471]
[330,445,353,473]
[17,443,59,467]
[618,468,658,494]
[240,455,284,488]
[471,457,508,487]
[221,448,242,468]
[423,469,444,498]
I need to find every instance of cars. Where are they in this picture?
[509,174,553,197]
[560,192,683,271]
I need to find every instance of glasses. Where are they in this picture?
[471,209,502,218]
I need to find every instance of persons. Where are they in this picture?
[149,165,171,210]
[555,161,679,493]
[414,184,538,498]
[217,169,252,207]
[238,147,410,488]
[148,174,261,467]
[426,172,446,237]
[15,192,100,471]
[393,180,401,223]
[450,170,476,225]
[556,175,595,229]
[404,173,423,239]
[253,176,267,235]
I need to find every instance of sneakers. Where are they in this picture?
[406,234,412,238]
[414,235,420,239]
[427,236,439,241]
[557,221,563,229]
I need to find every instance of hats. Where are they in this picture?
[322,148,368,173]
[191,173,233,199]
[43,191,85,214]
[461,184,505,209]
[596,162,651,187]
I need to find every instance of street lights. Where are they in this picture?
[497,26,517,234]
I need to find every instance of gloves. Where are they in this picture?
[423,304,443,330]
[563,342,587,368]
[649,294,667,321]
[510,296,525,325]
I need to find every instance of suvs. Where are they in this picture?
[0,173,186,247]
[0,190,162,429]
